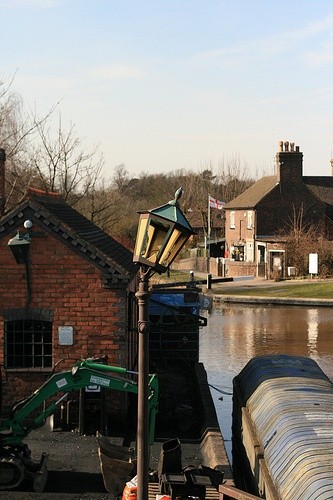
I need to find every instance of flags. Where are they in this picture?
[209,194,226,210]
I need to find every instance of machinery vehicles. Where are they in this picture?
[0,354,160,493]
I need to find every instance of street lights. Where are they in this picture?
[130,184,199,500]
